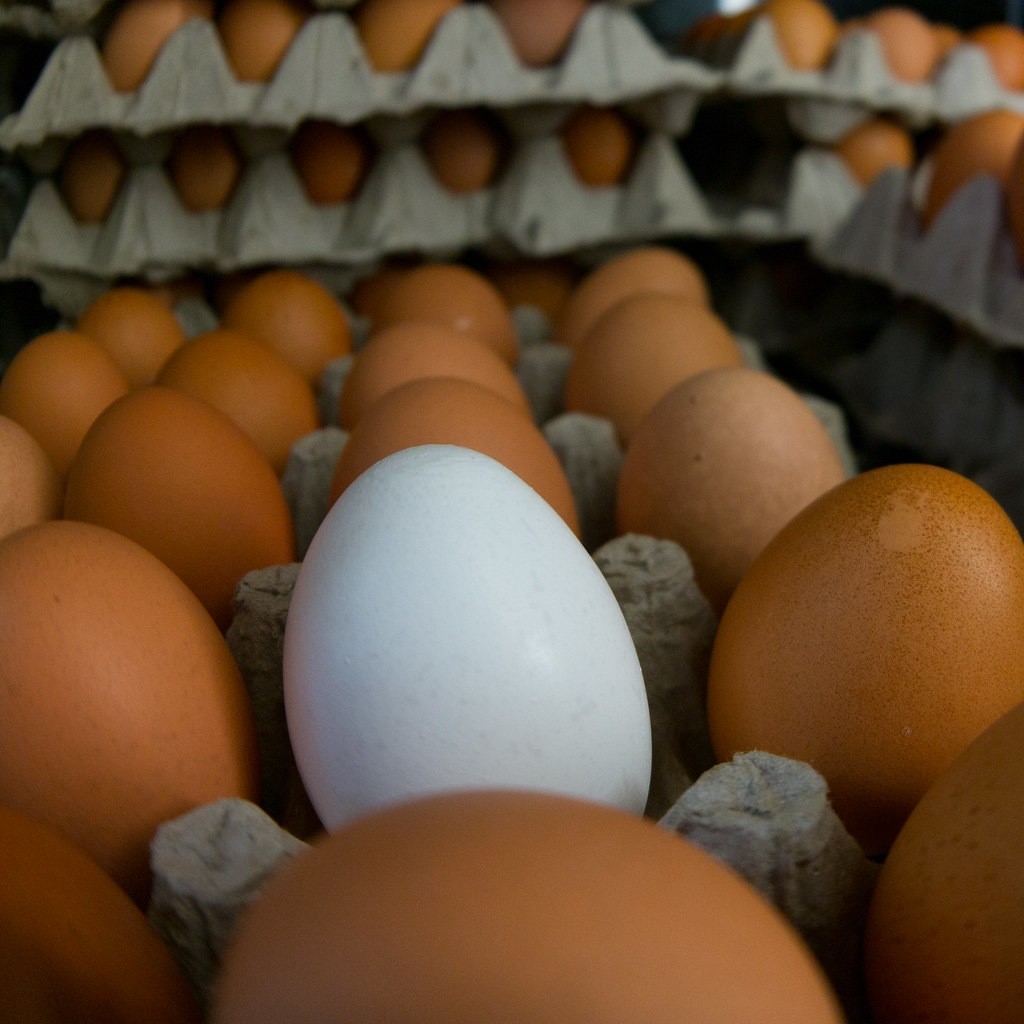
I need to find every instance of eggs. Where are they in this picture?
[0,0,1024,1024]
